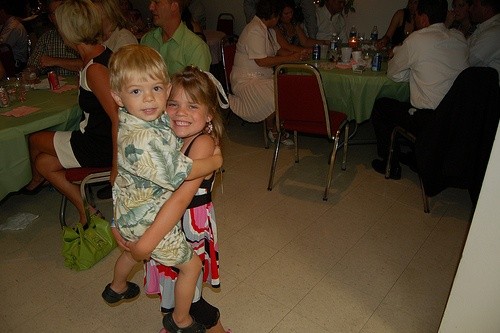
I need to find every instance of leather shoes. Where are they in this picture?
[370,158,403,180]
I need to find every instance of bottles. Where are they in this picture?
[328,33,338,62]
[146,18,151,31]
[371,25,380,41]
[350,25,357,38]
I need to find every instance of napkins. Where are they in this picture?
[0,106,41,118]
[49,84,79,94]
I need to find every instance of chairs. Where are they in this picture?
[221,34,269,149]
[386,66,500,213]
[216,13,234,38]
[60,166,112,229]
[267,64,348,204]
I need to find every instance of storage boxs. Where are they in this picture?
[353,66,366,73]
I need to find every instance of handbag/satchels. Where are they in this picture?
[63,215,118,271]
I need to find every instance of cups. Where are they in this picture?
[320,44,329,58]
[352,51,361,62]
[341,47,352,62]
[5,75,25,102]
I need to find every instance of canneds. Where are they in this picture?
[371,53,382,71]
[48,71,61,90]
[0,88,10,108]
[312,44,321,60]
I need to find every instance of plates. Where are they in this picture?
[336,64,351,68]
[25,81,43,88]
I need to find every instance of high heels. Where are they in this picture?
[267,127,295,145]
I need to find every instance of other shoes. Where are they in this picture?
[163,312,205,333]
[102,281,141,307]
[73,209,106,234]
[97,185,113,199]
[19,178,52,195]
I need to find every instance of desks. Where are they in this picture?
[285,58,410,144]
[0,75,82,200]
[206,28,227,65]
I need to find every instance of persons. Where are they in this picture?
[0,0,213,89]
[224,0,315,146]
[372,0,471,180]
[111,65,233,333]
[272,0,500,99]
[19,0,114,235]
[101,45,223,333]
[137,0,211,85]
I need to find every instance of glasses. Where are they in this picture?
[49,10,55,17]
